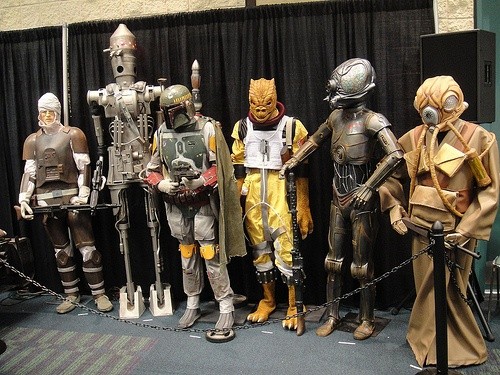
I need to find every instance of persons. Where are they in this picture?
[278,58,406,340]
[18,93,113,313]
[231,77,313,329]
[146,84,247,329]
[379,76,500,367]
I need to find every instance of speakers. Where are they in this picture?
[418,28,497,124]
